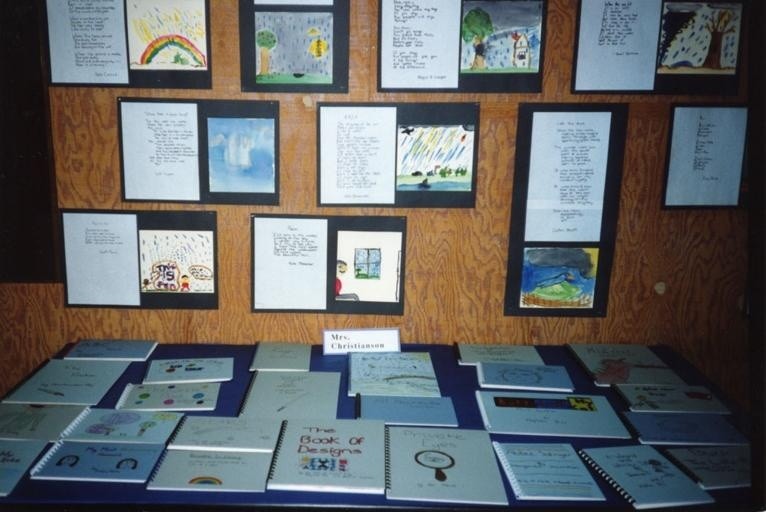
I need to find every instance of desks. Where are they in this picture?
[1,341,766,511]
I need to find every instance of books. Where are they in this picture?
[0,336,752,508]
